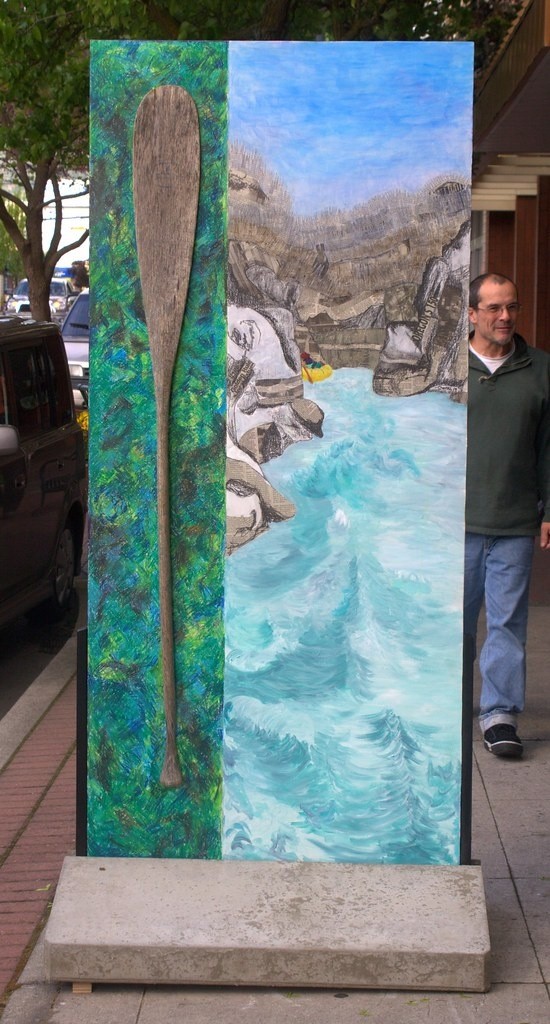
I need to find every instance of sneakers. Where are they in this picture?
[484,723,523,757]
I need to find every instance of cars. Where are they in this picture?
[0,268,88,411]
[0,318,87,632]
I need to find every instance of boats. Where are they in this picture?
[304,365,333,383]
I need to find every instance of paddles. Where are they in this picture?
[128,82,203,796]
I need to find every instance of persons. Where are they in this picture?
[72,259,90,292]
[463,273,550,757]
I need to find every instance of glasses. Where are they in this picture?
[476,303,525,316]
